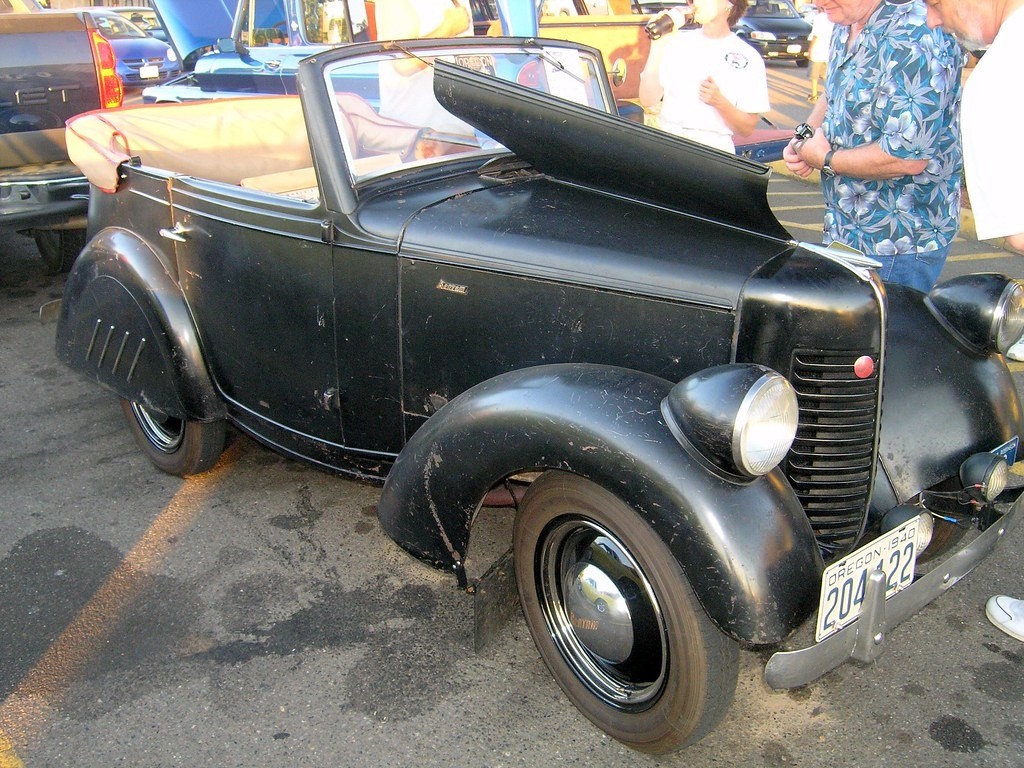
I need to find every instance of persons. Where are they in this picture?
[920,0,1024,643]
[805,1,837,104]
[373,0,476,162]
[960,48,988,88]
[639,0,769,157]
[781,0,964,296]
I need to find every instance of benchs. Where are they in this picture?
[65,93,480,207]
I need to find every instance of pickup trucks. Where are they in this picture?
[470,2,702,119]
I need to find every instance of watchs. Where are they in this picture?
[821,149,839,179]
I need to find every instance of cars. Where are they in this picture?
[142,1,475,105]
[107,7,167,41]
[734,0,816,68]
[85,10,184,93]
[0,1,125,273]
[36,34,1024,758]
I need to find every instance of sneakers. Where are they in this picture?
[985,595,1024,642]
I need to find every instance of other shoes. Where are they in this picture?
[808,94,818,104]
